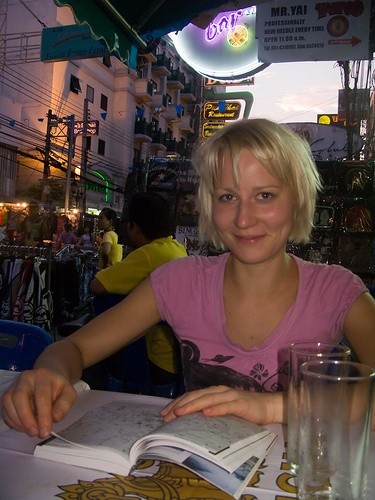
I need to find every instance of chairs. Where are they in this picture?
[0,319,54,373]
[82,319,187,400]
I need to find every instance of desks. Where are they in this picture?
[0,388,375,500]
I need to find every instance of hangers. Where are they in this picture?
[0,243,47,260]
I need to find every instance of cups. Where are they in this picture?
[296,358,375,500]
[288,341,352,477]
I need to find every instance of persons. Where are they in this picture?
[58,207,124,269]
[88,192,188,395]
[0,119,375,438]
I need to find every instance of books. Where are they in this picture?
[32,395,278,499]
